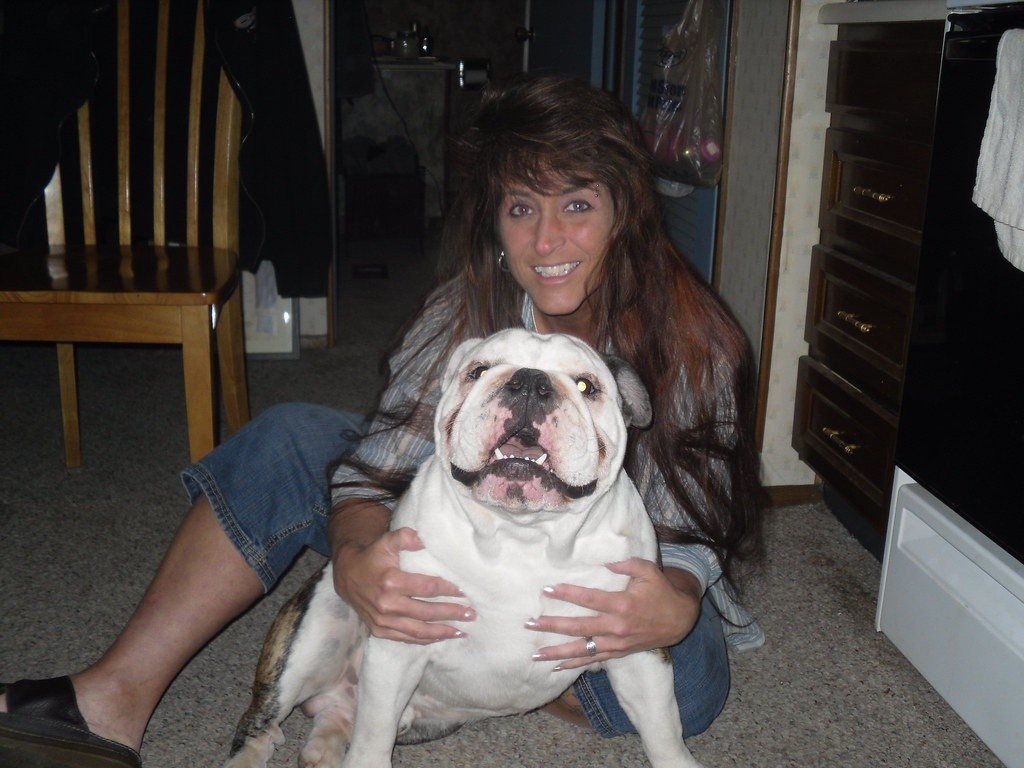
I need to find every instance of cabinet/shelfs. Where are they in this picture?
[344,57,459,232]
[789,0,1024,567]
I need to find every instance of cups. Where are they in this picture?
[419,37,433,56]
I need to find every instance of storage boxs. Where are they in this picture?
[447,68,478,202]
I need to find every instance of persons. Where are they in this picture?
[1,69,759,768]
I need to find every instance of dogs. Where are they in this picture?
[222,327,705,768]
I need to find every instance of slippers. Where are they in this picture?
[0,675,142,768]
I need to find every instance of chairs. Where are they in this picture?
[1,0,255,470]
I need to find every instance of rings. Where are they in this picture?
[586,636,598,657]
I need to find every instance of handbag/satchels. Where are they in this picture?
[637,0,723,188]
